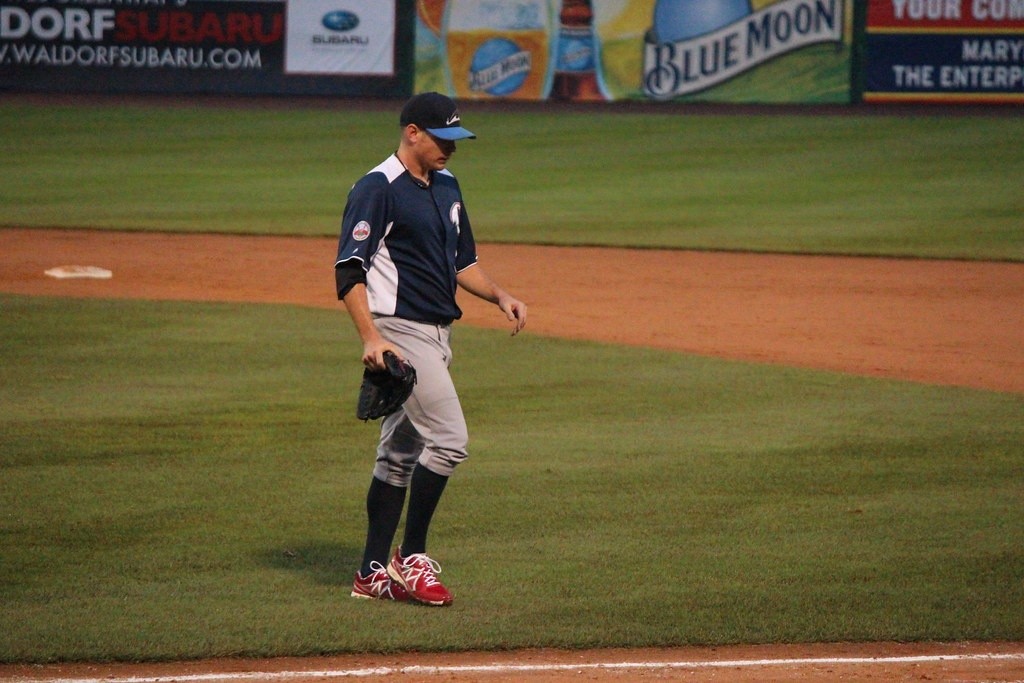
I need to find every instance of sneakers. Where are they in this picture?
[387,545,453,605]
[351,561,415,602]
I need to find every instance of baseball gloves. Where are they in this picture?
[355,350,418,429]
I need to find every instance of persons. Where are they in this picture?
[333,91,529,608]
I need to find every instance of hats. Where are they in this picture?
[399,91,477,140]
